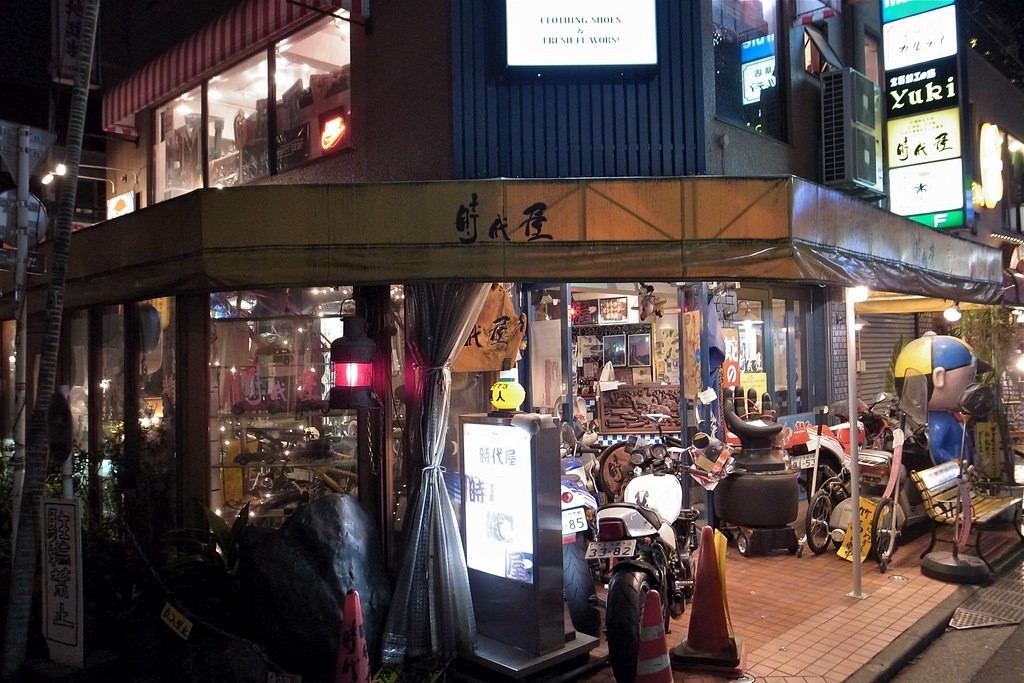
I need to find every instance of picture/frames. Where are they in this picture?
[572,296,651,367]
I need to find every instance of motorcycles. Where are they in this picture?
[561,411,696,682]
[228,418,358,545]
[152,495,373,683]
[782,367,942,563]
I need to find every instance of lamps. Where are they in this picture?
[943,298,963,322]
[37,156,137,194]
[724,300,765,326]
[315,295,385,414]
[530,286,552,306]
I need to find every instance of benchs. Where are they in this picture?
[911,456,1024,574]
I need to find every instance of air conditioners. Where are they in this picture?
[819,66,887,198]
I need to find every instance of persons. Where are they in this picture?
[895,331,993,480]
[723,386,785,469]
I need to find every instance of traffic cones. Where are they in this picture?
[333,590,370,683]
[635,588,674,683]
[669,525,747,678]
